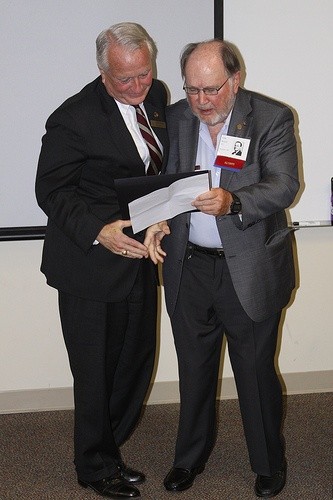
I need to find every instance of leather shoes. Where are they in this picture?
[77,473,141,500]
[163,463,205,491]
[254,460,287,497]
[116,463,145,484]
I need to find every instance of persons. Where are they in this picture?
[144,40,300,497]
[35,22,169,500]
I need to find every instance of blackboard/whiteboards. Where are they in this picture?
[1,0,333,245]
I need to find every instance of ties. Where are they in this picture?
[131,104,164,175]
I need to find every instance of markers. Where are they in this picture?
[293,221,321,226]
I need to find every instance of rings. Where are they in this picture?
[122,250,127,255]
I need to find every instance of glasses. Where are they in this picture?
[182,76,230,95]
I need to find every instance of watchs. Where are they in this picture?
[226,194,241,215]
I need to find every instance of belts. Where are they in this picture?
[191,243,227,258]
